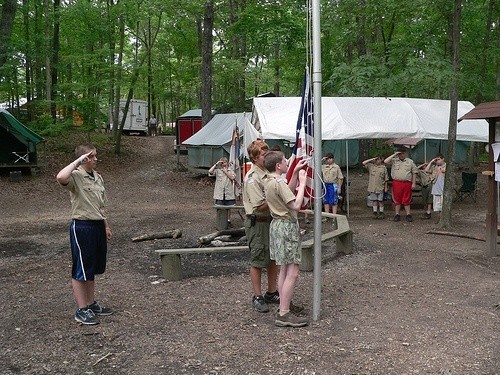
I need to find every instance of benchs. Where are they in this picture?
[155,204,353,280]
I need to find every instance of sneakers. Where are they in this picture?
[252,294,270,313]
[264,290,280,304]
[275,311,309,327]
[276,299,305,313]
[74,305,100,325]
[88,302,114,315]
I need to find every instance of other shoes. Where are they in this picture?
[424,212,431,219]
[373,212,378,220]
[378,212,385,220]
[394,214,400,222]
[406,214,413,222]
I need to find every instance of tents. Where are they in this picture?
[181,113,253,146]
[248,94,491,183]
[1,109,47,166]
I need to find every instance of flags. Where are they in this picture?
[279,64,319,210]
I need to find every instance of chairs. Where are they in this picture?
[453,172,477,203]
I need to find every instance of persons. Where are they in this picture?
[262,151,312,327]
[426,153,446,223]
[320,152,343,224]
[209,157,237,228]
[56,143,114,325]
[243,140,284,312]
[149,115,157,136]
[416,161,434,219]
[384,146,418,223]
[362,154,390,219]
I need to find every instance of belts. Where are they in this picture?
[247,215,272,222]
